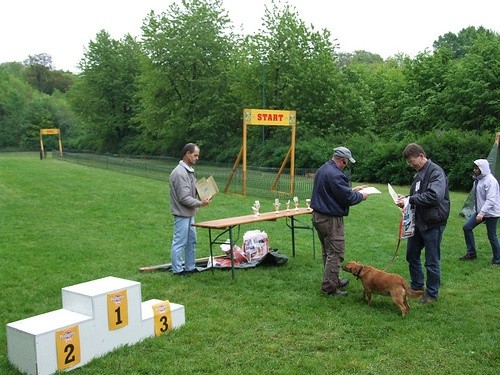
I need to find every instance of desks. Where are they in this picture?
[191,208,315,280]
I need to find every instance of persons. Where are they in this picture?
[169,143,210,275]
[396,143,451,302]
[460,159,500,264]
[308,146,368,296]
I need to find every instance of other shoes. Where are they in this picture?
[417,295,437,303]
[491,261,500,265]
[321,278,350,297]
[175,271,189,276]
[459,251,477,259]
[186,268,199,273]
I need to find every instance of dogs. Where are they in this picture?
[340,259,424,317]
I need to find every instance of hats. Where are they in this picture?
[333,147,356,163]
